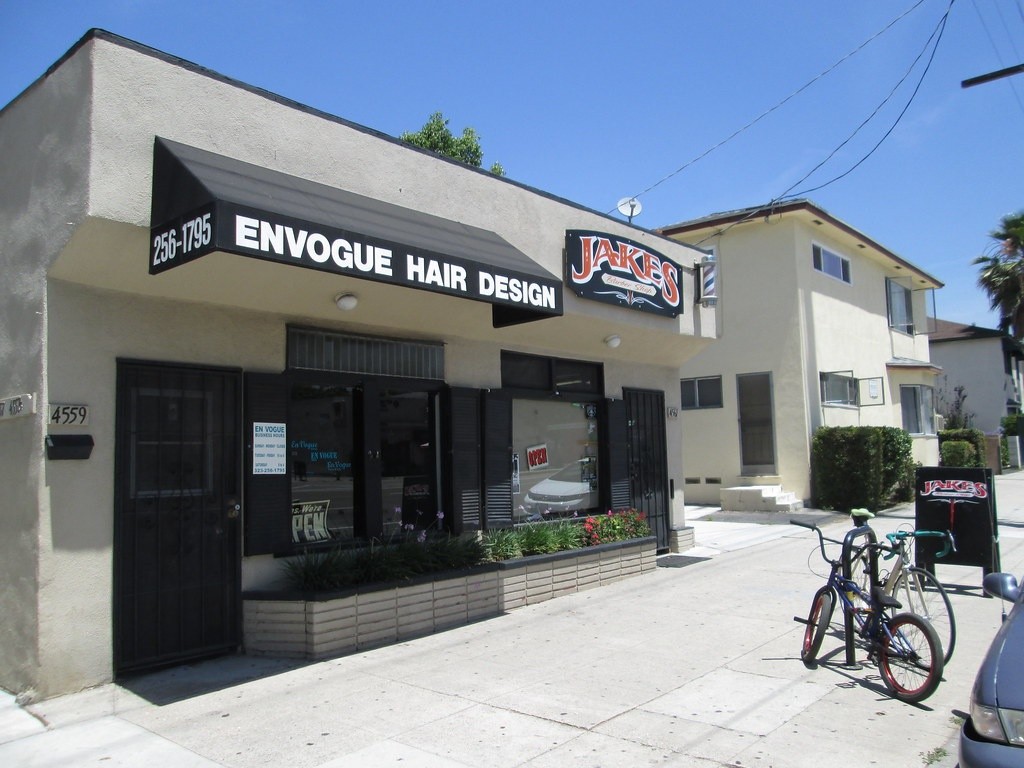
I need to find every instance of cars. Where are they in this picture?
[521,456,599,516]
[958,570,1024,768]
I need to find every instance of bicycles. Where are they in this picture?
[848,506,959,666]
[787,517,946,702]
